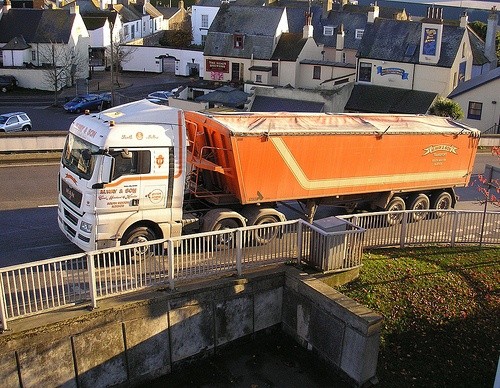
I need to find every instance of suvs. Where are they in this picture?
[0,74,17,94]
[0,112,33,132]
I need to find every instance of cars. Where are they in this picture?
[62,91,125,114]
[146,88,179,104]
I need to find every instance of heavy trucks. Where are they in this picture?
[56,98,482,264]
